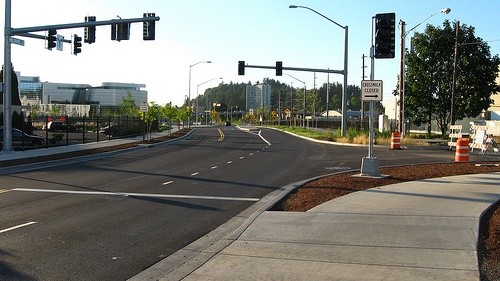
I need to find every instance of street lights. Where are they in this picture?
[196,77,224,125]
[289,5,349,138]
[187,61,212,129]
[398,7,451,134]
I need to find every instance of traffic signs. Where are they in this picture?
[361,80,383,102]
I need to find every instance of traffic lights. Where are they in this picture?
[48,29,57,50]
[74,34,82,55]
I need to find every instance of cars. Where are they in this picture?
[99,124,139,137]
[0,126,46,147]
[225,122,231,126]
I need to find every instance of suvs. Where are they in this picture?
[47,121,63,131]
